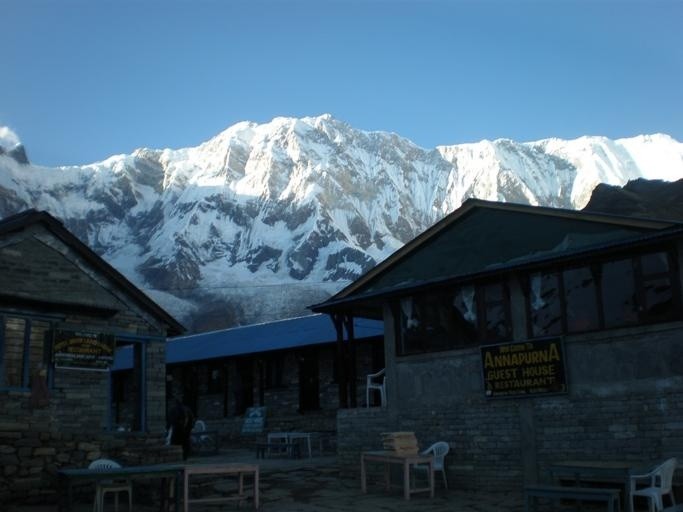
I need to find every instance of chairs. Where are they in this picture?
[422,441,450,489]
[366,368,386,408]
[87,459,132,511]
[629,457,678,512]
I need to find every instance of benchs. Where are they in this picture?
[523,482,621,512]
[256,442,300,459]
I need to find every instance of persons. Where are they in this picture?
[169,398,200,455]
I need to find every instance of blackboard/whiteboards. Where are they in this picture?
[242,407,266,434]
[480,335,569,401]
[51,327,115,371]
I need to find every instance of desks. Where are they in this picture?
[267,432,322,464]
[360,451,435,501]
[40,462,260,512]
[553,460,636,512]
